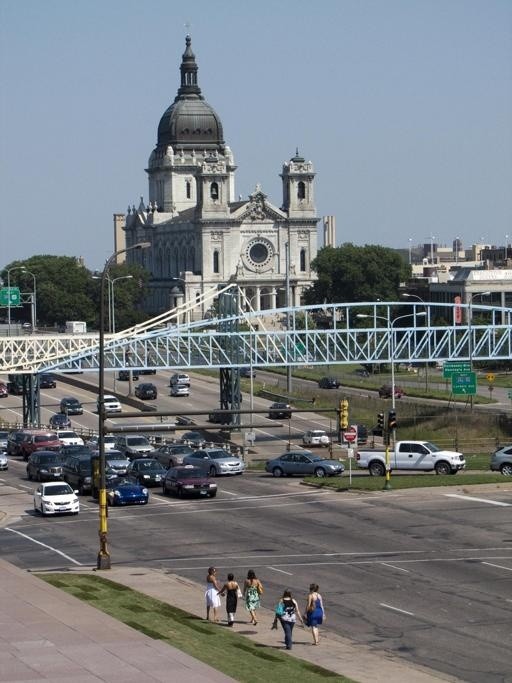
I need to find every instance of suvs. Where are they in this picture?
[490,444,512,476]
[94,394,124,415]
[337,421,371,446]
[103,337,159,381]
[302,428,333,448]
[59,395,84,414]
[265,401,295,420]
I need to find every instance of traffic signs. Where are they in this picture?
[0,286,20,306]
[451,371,478,395]
[442,361,473,377]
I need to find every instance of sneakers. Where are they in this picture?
[251,620,257,625]
[228,620,233,626]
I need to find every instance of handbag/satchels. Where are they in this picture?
[276,602,284,617]
[257,584,263,594]
[236,588,242,598]
[306,600,315,612]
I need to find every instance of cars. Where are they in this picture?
[0,425,247,514]
[314,375,342,389]
[264,448,348,479]
[206,406,230,424]
[21,322,31,329]
[0,344,192,398]
[375,382,404,399]
[46,412,75,428]
[241,342,320,378]
[349,368,374,379]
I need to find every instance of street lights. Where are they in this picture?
[355,310,431,412]
[90,274,134,335]
[219,288,281,426]
[469,290,493,371]
[21,269,38,328]
[400,233,510,266]
[7,265,29,335]
[173,276,192,329]
[402,292,429,352]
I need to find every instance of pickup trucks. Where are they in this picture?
[352,438,467,477]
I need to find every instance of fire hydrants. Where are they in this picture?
[97,235,155,573]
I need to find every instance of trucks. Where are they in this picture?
[63,320,86,333]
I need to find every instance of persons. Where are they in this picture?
[243,569,263,625]
[204,567,225,620]
[216,572,239,626]
[306,583,327,645]
[276,589,303,648]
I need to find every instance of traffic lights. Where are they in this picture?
[378,412,385,429]
[388,410,398,432]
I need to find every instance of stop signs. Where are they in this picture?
[342,425,357,443]
